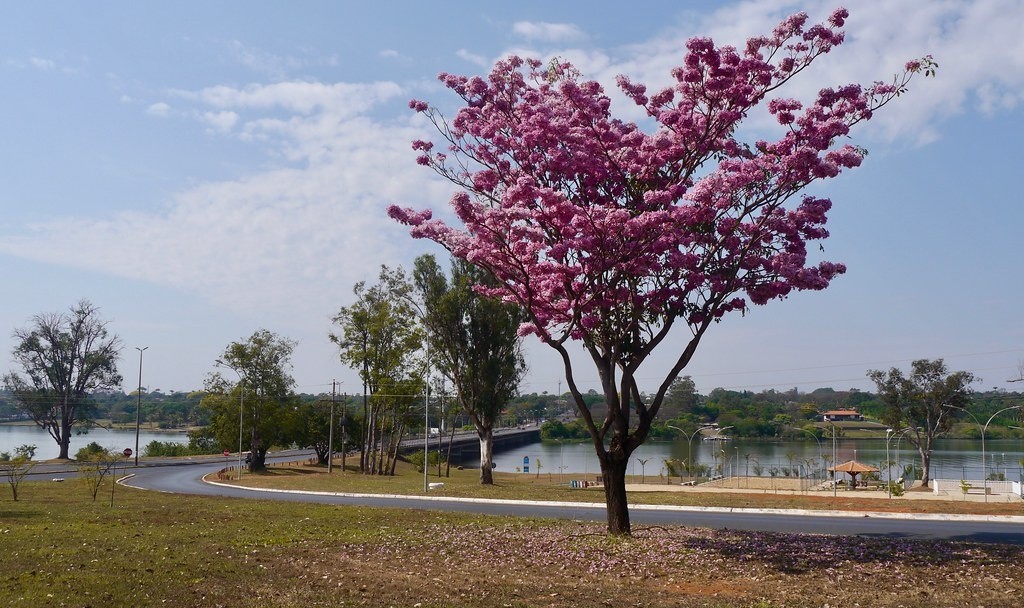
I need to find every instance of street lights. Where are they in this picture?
[134,347,149,466]
[794,427,823,487]
[667,425,710,483]
[712,426,734,483]
[554,439,563,484]
[214,359,243,480]
[579,443,587,481]
[896,426,923,481]
[389,288,430,492]
[859,427,911,500]
[942,403,1021,504]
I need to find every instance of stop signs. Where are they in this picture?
[124,447,132,456]
[224,450,230,456]
